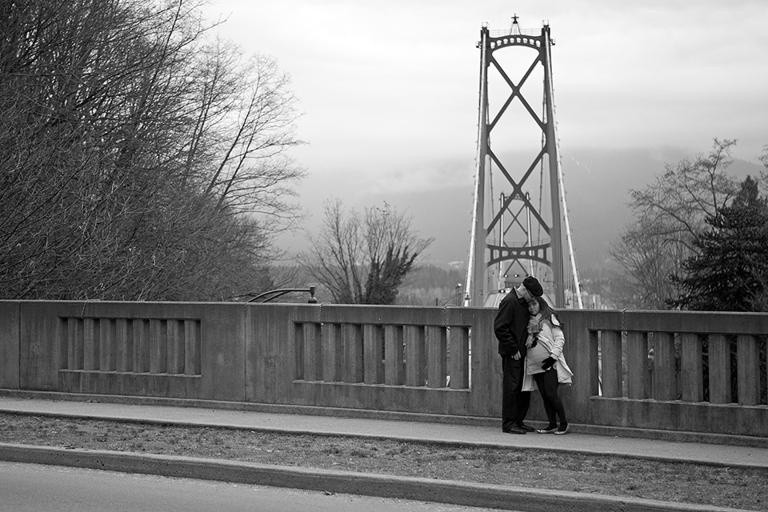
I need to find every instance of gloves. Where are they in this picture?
[541,355,557,370]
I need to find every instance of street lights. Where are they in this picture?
[454,283,462,306]
[248,286,318,304]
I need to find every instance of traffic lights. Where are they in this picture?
[498,289,506,293]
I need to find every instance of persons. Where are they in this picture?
[493,276,545,435]
[521,297,574,436]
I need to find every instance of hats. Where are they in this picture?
[523,276,544,296]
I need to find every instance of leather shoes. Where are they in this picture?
[504,422,535,434]
[536,421,570,435]
